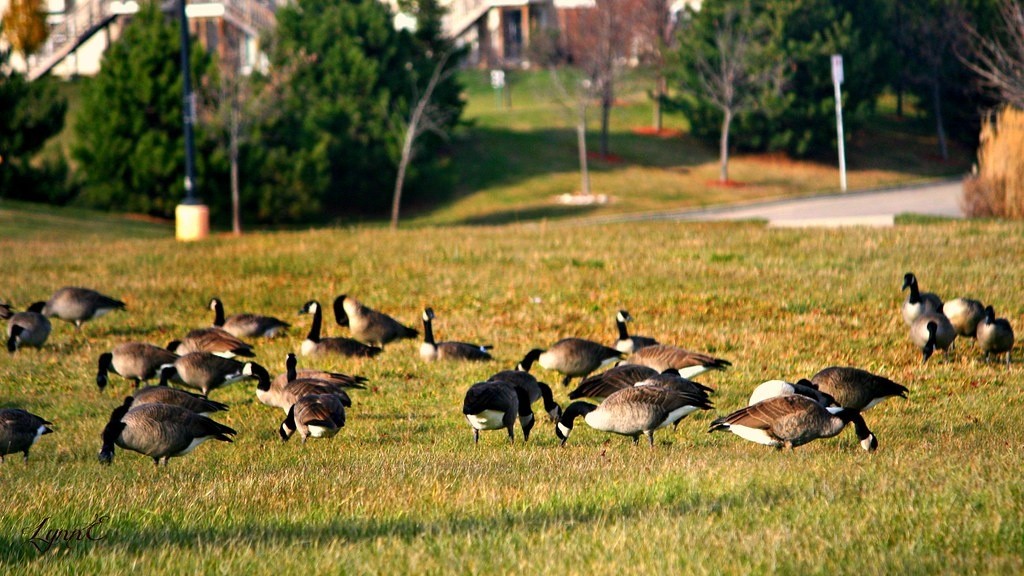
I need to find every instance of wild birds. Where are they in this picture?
[0,273,1014,478]
